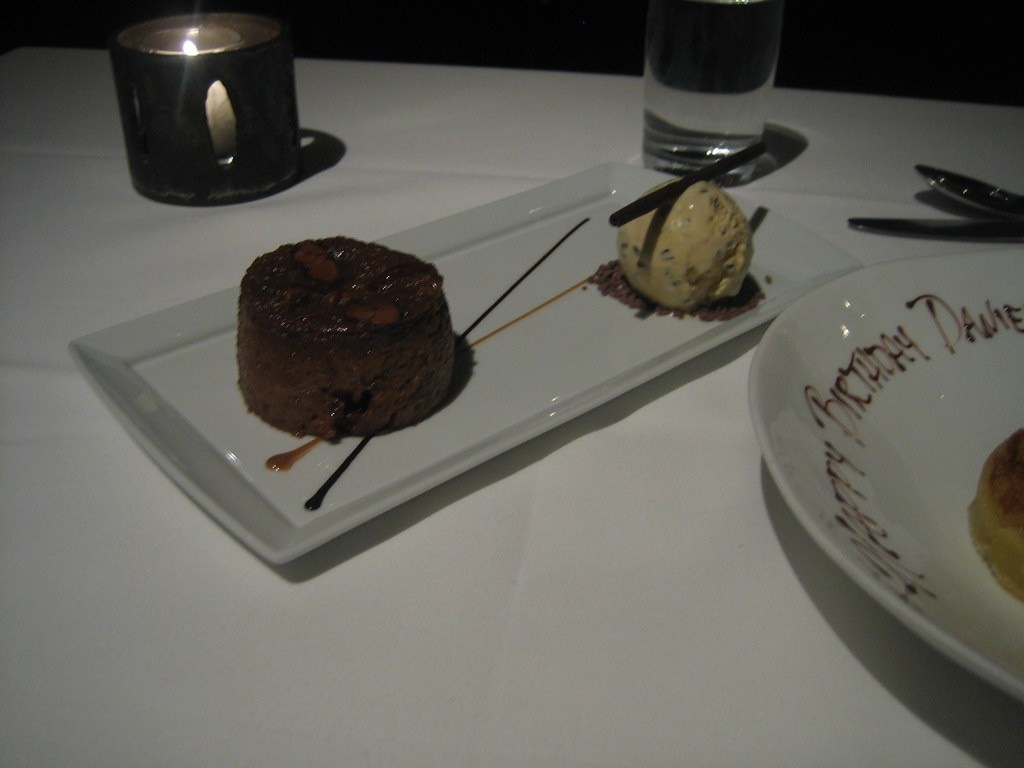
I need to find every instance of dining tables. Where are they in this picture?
[0,44,1024,768]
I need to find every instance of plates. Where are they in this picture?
[748,247,1024,704]
[66,159,864,564]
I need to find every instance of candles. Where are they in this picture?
[182,40,237,157]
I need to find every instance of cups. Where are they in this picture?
[639,0,784,183]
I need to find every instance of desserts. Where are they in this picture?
[966,425,1024,604]
[235,235,454,442]
[608,143,771,313]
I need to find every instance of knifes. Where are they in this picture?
[846,164,1024,244]
